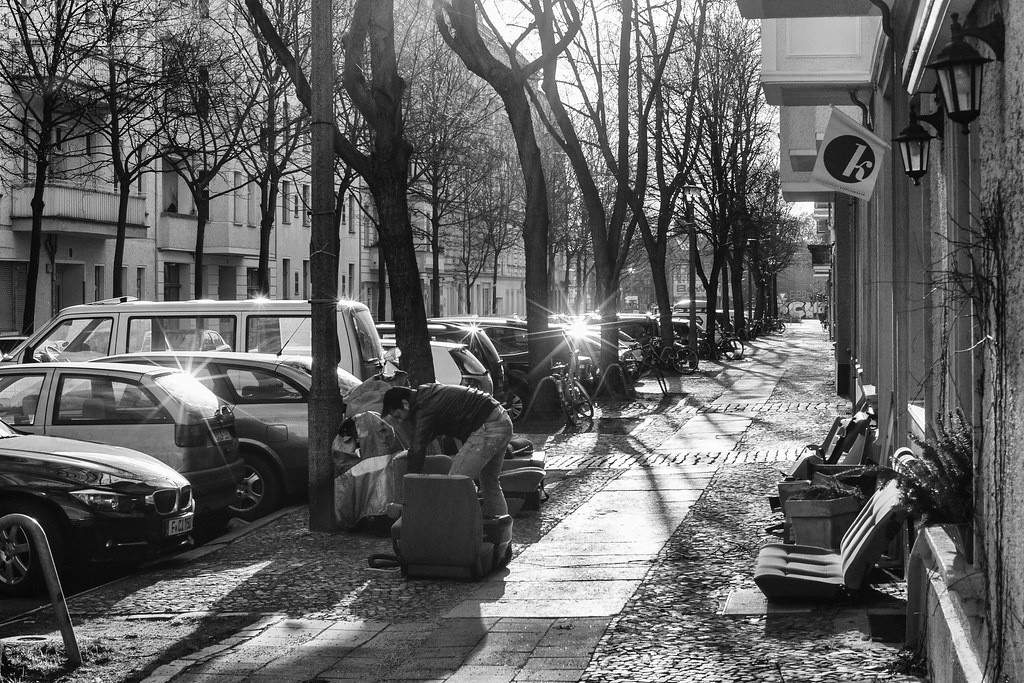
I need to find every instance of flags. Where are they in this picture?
[810,106,887,202]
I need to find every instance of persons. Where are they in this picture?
[381,384,514,568]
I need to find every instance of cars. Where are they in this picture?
[1,351,364,521]
[138,326,234,358]
[0,419,194,598]
[253,300,759,423]
[0,362,237,541]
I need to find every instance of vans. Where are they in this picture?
[0,296,385,399]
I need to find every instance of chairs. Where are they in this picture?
[82,398,106,419]
[22,395,40,417]
[752,446,919,607]
[442,436,546,516]
[392,474,513,582]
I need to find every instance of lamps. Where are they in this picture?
[923,11,1006,137]
[891,105,943,187]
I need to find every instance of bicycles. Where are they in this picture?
[622,315,786,379]
[551,350,594,426]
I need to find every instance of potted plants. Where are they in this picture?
[785,485,869,550]
